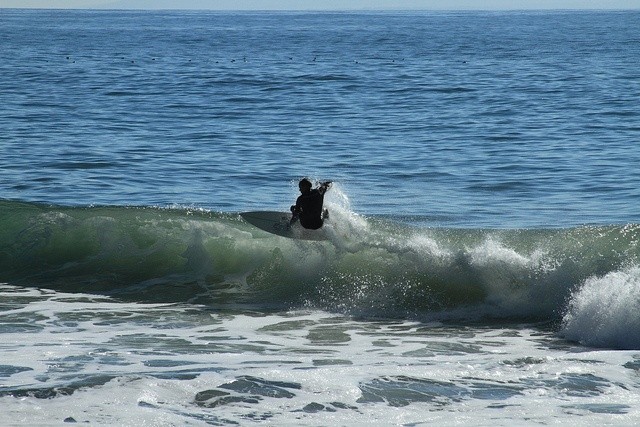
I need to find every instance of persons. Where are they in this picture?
[284,177,332,230]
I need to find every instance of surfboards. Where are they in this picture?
[239,211,334,240]
